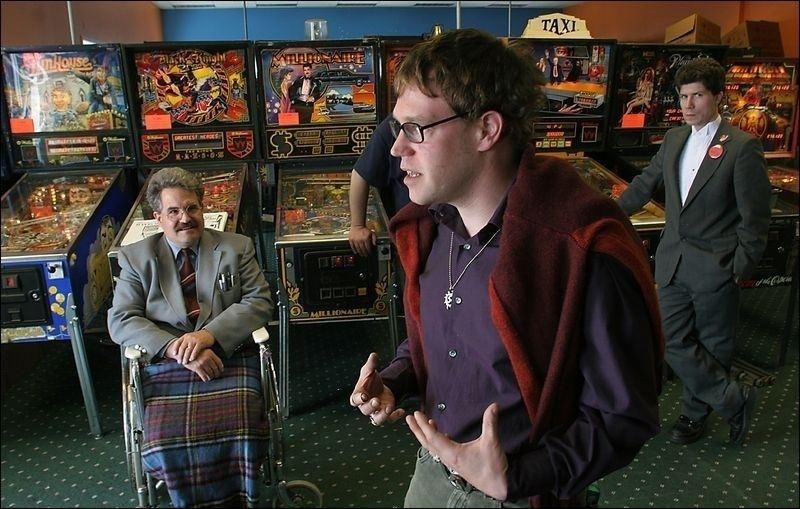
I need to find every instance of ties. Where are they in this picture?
[179,249,200,327]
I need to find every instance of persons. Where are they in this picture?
[104,164,277,509]
[286,62,321,124]
[618,68,655,124]
[278,66,294,113]
[5,65,113,130]
[348,111,410,259]
[613,53,773,448]
[347,25,666,509]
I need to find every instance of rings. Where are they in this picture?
[433,454,442,465]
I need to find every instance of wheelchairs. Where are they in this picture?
[119,313,323,507]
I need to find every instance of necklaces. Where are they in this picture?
[442,230,500,311]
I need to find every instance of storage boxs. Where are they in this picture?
[664,12,722,45]
[719,19,784,57]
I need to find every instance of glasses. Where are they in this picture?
[388,112,468,143]
[160,202,202,222]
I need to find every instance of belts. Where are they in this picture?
[439,462,480,493]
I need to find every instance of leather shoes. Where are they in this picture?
[728,383,758,447]
[669,405,713,445]
